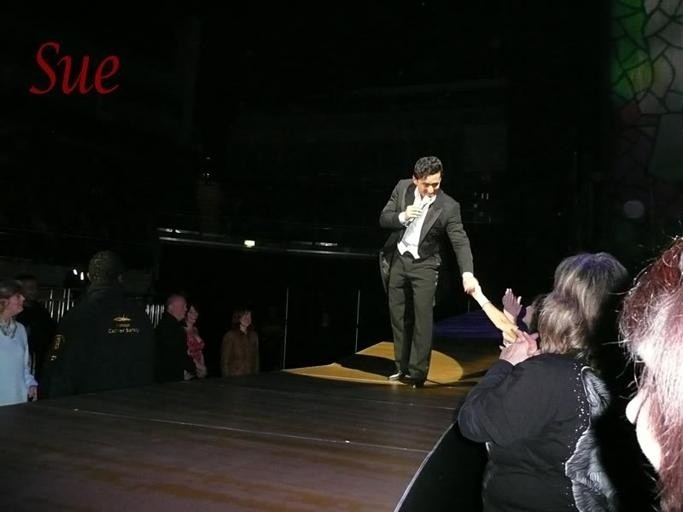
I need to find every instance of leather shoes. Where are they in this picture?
[389,373,425,388]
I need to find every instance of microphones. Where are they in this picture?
[404,195,430,228]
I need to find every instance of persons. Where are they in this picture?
[0,250,209,408]
[315,305,340,360]
[259,305,283,371]
[378,156,476,387]
[219,307,260,379]
